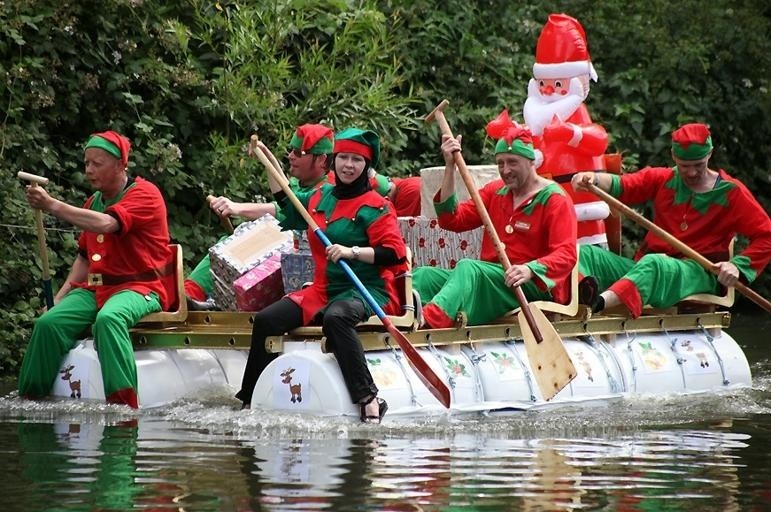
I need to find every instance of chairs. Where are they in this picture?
[133,236,744,332]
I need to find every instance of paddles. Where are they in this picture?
[426,98,577,402]
[251,136,451,409]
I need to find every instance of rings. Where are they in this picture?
[332,253,336,257]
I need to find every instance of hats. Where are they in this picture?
[289,123,334,157]
[495,129,534,162]
[334,127,380,180]
[84,131,133,167]
[671,123,713,162]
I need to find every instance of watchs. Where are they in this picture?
[350,243,361,260]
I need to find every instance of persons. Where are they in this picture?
[15,129,173,417]
[18,425,140,512]
[412,126,578,329]
[364,165,421,215]
[231,127,407,427]
[485,13,611,252]
[569,122,771,321]
[186,122,334,310]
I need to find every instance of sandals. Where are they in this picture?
[360,396,388,423]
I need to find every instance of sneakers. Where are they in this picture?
[578,275,600,309]
[412,288,426,328]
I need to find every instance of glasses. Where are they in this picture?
[286,144,303,157]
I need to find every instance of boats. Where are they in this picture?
[40,303,758,426]
[50,410,750,511]
